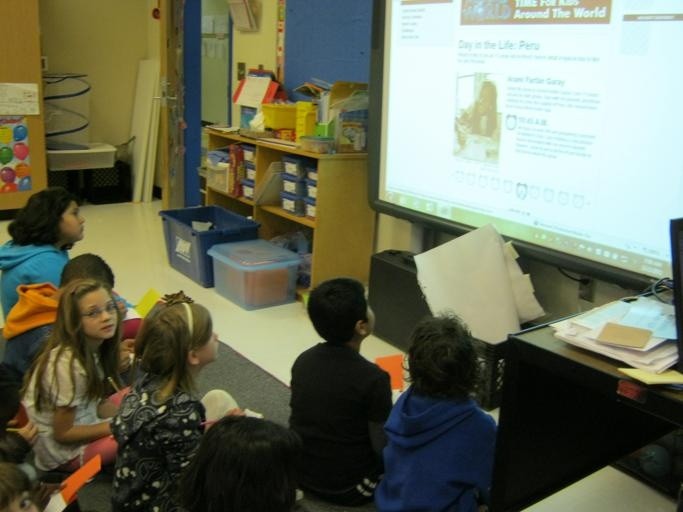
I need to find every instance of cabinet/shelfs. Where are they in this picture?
[202,122,376,308]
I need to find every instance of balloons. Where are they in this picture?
[13,143,30,161]
[0,166,16,183]
[1,183,19,193]
[0,147,13,164]
[17,176,33,190]
[13,125,28,141]
[0,126,13,144]
[15,162,31,177]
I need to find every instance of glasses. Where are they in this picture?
[78,303,118,318]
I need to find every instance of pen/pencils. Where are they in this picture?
[201,419,222,426]
[108,376,124,390]
[6,428,48,431]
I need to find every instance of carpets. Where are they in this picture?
[75,341,378,510]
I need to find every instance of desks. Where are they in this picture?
[490,287,682,512]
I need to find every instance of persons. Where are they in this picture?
[288,276,395,507]
[1,253,129,372]
[1,188,85,323]
[1,362,39,461]
[110,289,246,511]
[180,414,300,512]
[372,312,498,512]
[0,461,41,512]
[22,278,139,481]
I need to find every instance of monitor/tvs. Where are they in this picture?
[366,0,683,294]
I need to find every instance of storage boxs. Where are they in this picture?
[300,197,316,219]
[205,158,231,196]
[243,163,257,184]
[279,192,303,215]
[240,144,256,164]
[240,179,253,201]
[159,205,261,288]
[282,172,305,194]
[281,154,311,178]
[303,178,317,200]
[303,166,318,184]
[207,239,303,313]
